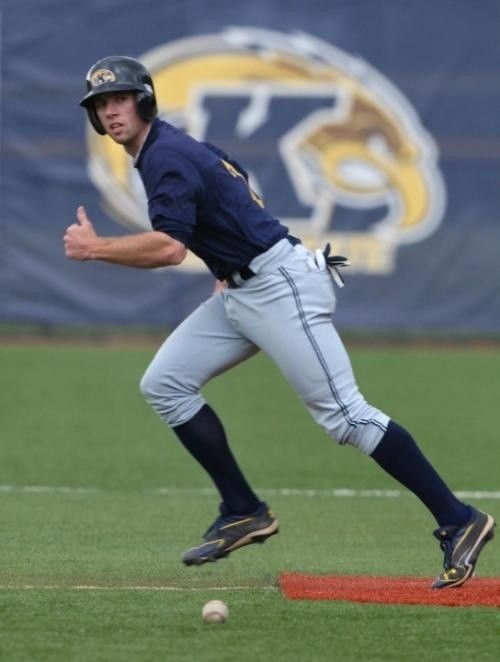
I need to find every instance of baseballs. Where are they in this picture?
[201,599,229,625]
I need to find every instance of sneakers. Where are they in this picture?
[181,502,280,567]
[431,506,495,590]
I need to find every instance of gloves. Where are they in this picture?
[306,243,350,287]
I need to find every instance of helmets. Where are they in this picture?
[78,56,157,135]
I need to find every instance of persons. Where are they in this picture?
[62,55,498,589]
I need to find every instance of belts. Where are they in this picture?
[226,234,298,288]
